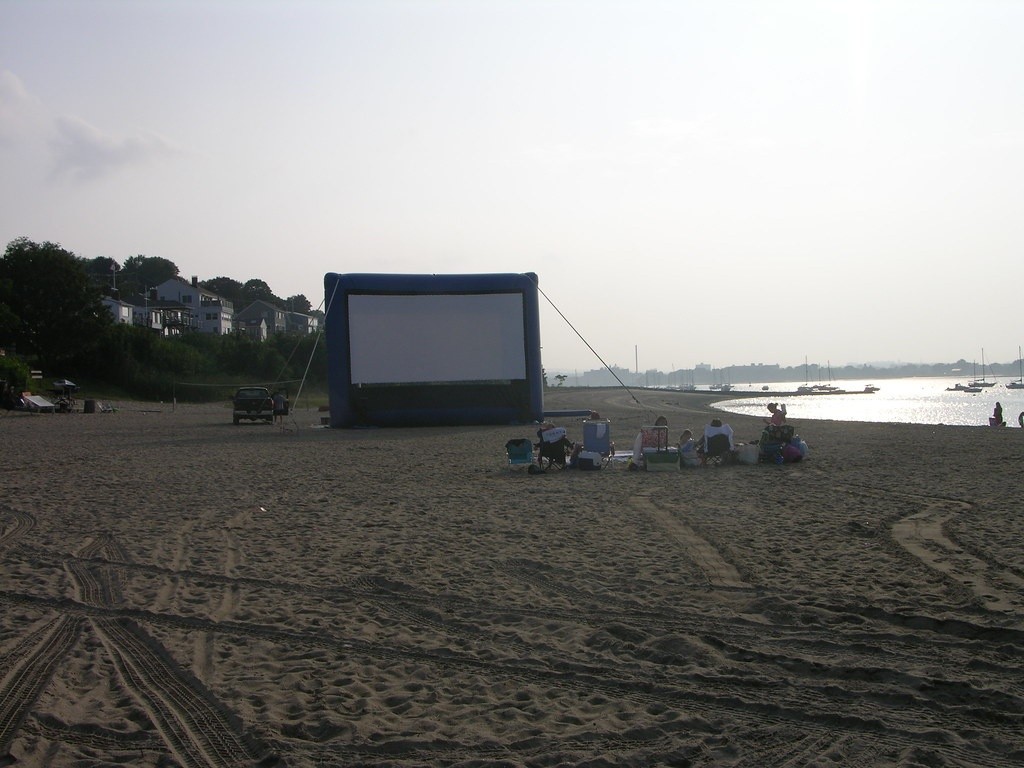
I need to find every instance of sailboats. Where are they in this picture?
[968,347,999,387]
[652,362,735,392]
[796,354,838,392]
[1006,346,1024,389]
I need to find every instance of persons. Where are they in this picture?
[676,429,698,453]
[763,403,787,426]
[1019,412,1024,427]
[993,403,1002,426]
[591,412,600,420]
[629,417,667,469]
[534,423,573,464]
[273,392,289,424]
[697,418,722,452]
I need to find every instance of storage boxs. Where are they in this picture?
[578,452,601,469]
[643,447,680,471]
[320,417,330,424]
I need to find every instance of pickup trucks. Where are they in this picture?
[229,386,291,425]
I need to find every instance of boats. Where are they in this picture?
[943,383,969,391]
[964,388,983,393]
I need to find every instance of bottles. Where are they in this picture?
[627,457,633,465]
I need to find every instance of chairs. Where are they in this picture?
[697,424,731,467]
[59,400,83,413]
[628,426,669,471]
[735,425,795,464]
[97,400,120,413]
[534,427,569,471]
[581,418,615,469]
[506,439,533,472]
